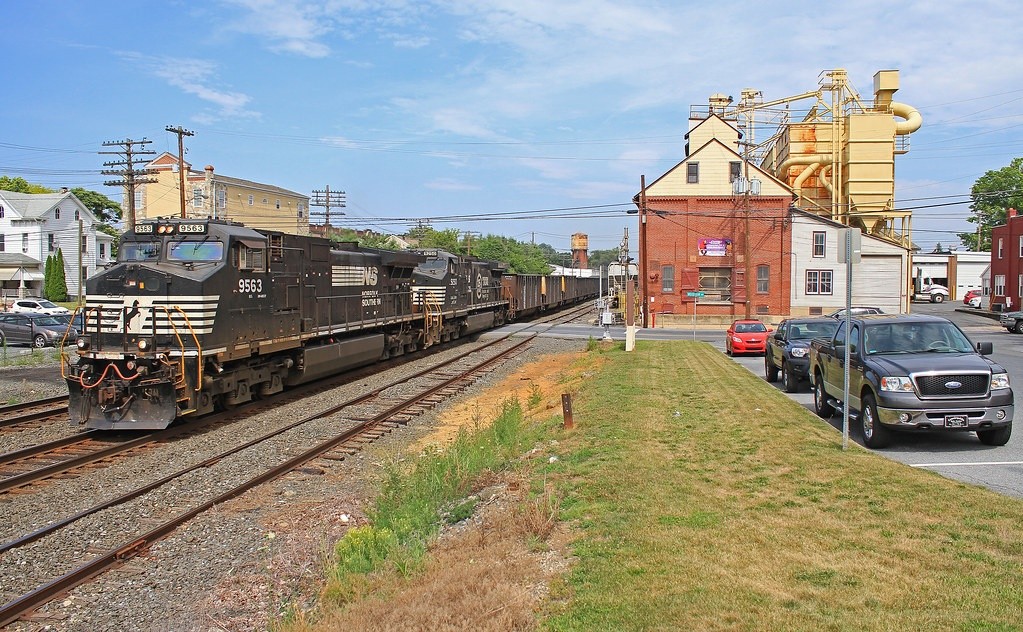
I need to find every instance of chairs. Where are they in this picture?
[913,325,942,351]
[790,326,801,338]
[819,327,831,337]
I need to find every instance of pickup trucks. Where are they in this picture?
[765,317,840,393]
[808,316,1014,448]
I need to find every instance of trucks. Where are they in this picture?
[914,267,949,303]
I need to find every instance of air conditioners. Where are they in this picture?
[22,247,28,253]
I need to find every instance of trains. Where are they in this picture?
[60,216,639,429]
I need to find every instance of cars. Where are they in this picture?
[963,290,981,309]
[50,314,84,334]
[726,319,773,357]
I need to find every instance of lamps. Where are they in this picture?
[238,194,242,196]
[288,202,291,204]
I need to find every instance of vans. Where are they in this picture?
[0,312,78,349]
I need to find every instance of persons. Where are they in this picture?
[699,241,707,254]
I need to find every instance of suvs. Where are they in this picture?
[1000,311,1023,334]
[821,307,887,321]
[10,299,70,315]
